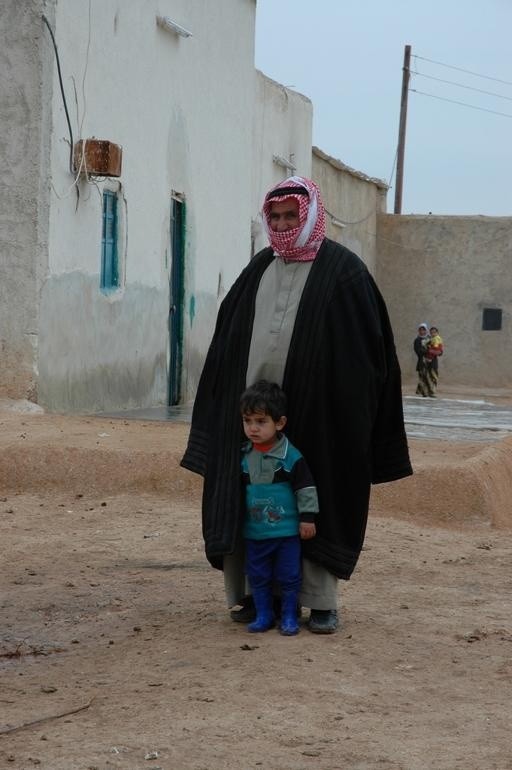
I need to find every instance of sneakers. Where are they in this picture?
[309,608,338,634]
[423,392,438,398]
[229,597,301,622]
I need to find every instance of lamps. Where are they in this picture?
[156,15,193,40]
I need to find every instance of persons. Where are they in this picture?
[425,327,443,363]
[229,380,318,637]
[177,176,414,638]
[411,322,444,399]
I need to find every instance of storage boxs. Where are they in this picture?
[75,139,123,178]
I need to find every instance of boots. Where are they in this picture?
[248,594,275,632]
[280,587,300,636]
